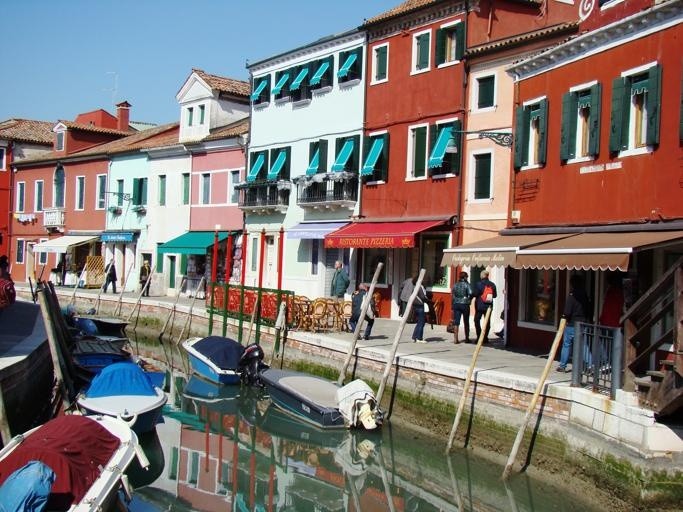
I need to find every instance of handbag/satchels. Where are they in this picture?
[360,295,375,319]
[424,302,429,312]
[447,321,454,333]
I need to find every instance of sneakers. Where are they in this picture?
[412,339,427,343]
[554,367,565,373]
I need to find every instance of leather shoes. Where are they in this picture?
[357,336,369,340]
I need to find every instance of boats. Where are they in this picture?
[0,415,140,512]
[182,336,270,385]
[67,313,168,433]
[258,366,385,431]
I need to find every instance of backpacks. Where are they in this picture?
[479,281,494,303]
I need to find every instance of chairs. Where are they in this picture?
[291,295,353,334]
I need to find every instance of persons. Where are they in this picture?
[349,282,375,340]
[555,274,595,376]
[451,271,472,344]
[102,258,117,294]
[399,278,415,317]
[330,260,349,330]
[0,254,16,311]
[138,259,150,297]
[471,269,497,343]
[410,276,427,343]
[597,285,624,365]
[494,309,504,338]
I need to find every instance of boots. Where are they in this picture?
[464,327,472,343]
[454,325,460,344]
